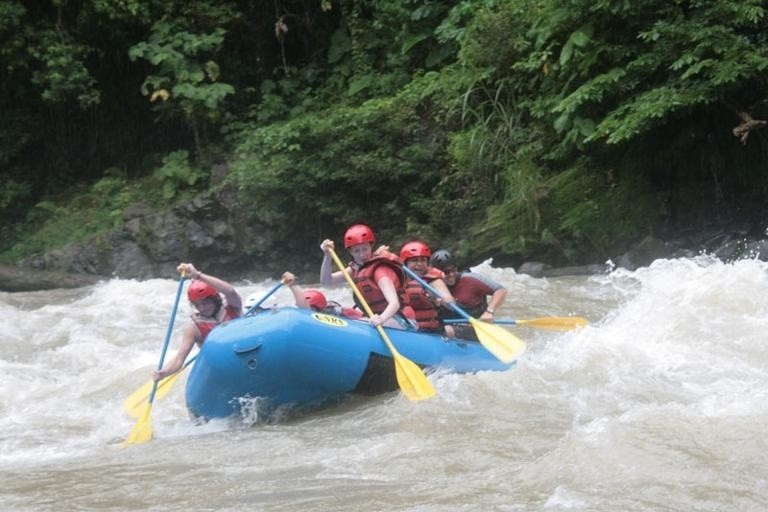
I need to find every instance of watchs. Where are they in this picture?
[486,307,496,314]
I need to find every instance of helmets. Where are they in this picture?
[399,240,431,267]
[244,291,279,310]
[295,291,327,312]
[429,249,458,273]
[187,278,218,302]
[343,223,376,250]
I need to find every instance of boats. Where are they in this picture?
[183,306,522,416]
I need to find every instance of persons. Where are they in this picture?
[282,271,327,311]
[151,262,242,382]
[373,241,456,338]
[244,290,279,314]
[320,224,419,333]
[428,249,507,344]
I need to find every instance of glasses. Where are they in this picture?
[443,265,457,275]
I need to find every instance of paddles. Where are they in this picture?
[129,270,188,444]
[401,263,528,364]
[328,242,436,402]
[123,279,286,418]
[443,316,590,333]
